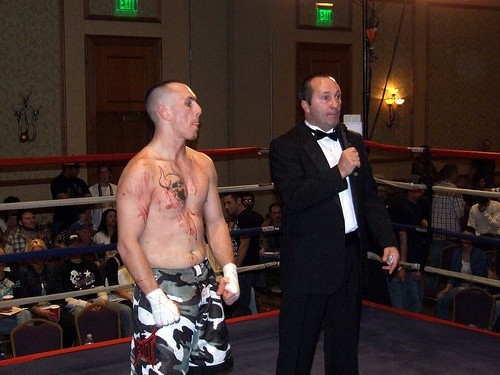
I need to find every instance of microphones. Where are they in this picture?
[335,123,359,177]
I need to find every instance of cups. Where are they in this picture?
[49,305,60,321]
[2,295,14,312]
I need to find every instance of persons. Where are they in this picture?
[116,81,241,375]
[223,191,280,319]
[0,162,137,338]
[368,139,500,331]
[269,74,400,375]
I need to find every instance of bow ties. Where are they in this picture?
[308,126,338,142]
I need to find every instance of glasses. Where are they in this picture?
[244,199,252,203]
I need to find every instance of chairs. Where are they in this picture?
[74,303,122,346]
[439,242,462,270]
[454,287,496,332]
[10,317,64,359]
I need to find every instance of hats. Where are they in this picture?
[65,233,82,245]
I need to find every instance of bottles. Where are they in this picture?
[85,334,94,345]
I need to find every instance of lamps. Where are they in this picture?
[384,86,406,129]
[10,88,43,146]
